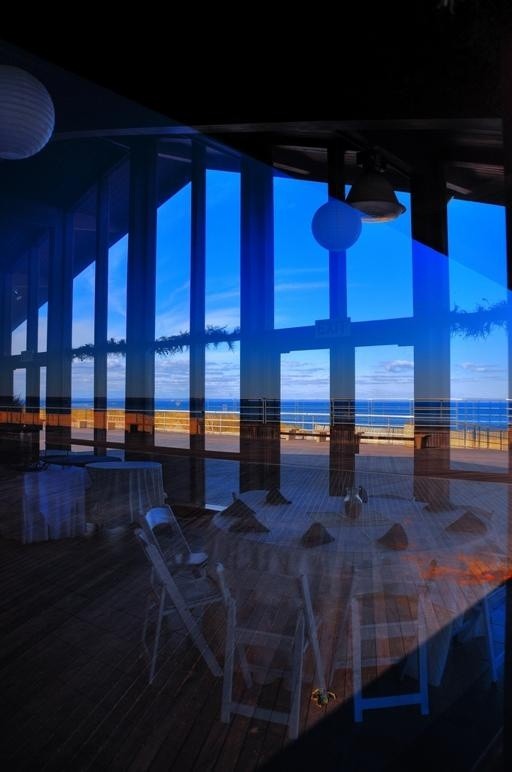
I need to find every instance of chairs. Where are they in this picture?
[137,503,227,684]
[214,538,505,740]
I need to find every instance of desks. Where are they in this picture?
[211,488,498,710]
[1,451,162,544]
